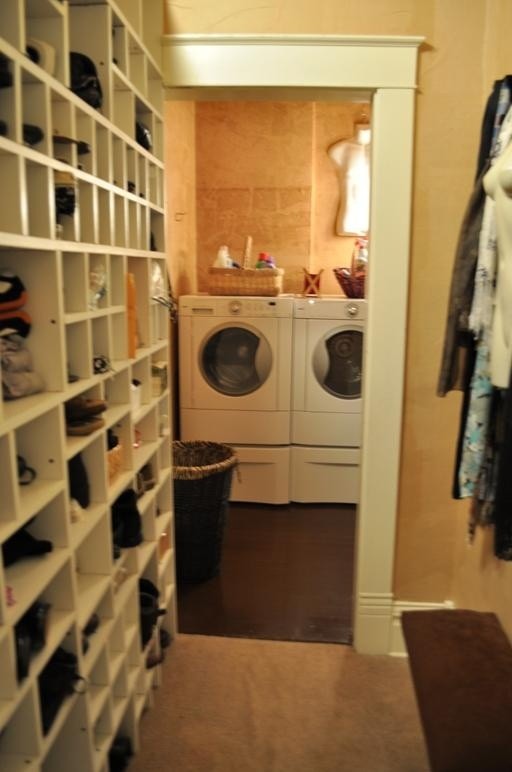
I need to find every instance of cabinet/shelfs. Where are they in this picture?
[0,2,186,772]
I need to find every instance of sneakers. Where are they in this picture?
[66,416,104,436]
[65,394,107,420]
[0,274,29,311]
[0,312,31,337]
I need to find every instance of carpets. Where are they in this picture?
[127,632,433,772]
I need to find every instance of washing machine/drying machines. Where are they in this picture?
[292,294,369,503]
[178,293,293,506]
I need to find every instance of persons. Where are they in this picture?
[328,115,370,238]
[477,134,512,388]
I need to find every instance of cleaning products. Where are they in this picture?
[255,252,269,269]
[212,244,232,269]
[355,239,368,272]
[263,256,277,270]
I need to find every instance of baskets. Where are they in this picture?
[333,242,366,299]
[152,360,167,397]
[206,235,284,296]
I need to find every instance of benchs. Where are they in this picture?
[398,604,511,772]
